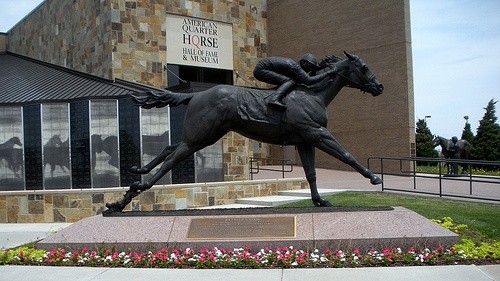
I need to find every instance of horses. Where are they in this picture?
[141,132,170,159]
[92,135,102,170]
[103,51,383,215]
[0,136,23,177]
[43,135,63,176]
[432,134,471,177]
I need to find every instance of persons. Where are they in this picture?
[447,136,462,176]
[253,53,337,110]
